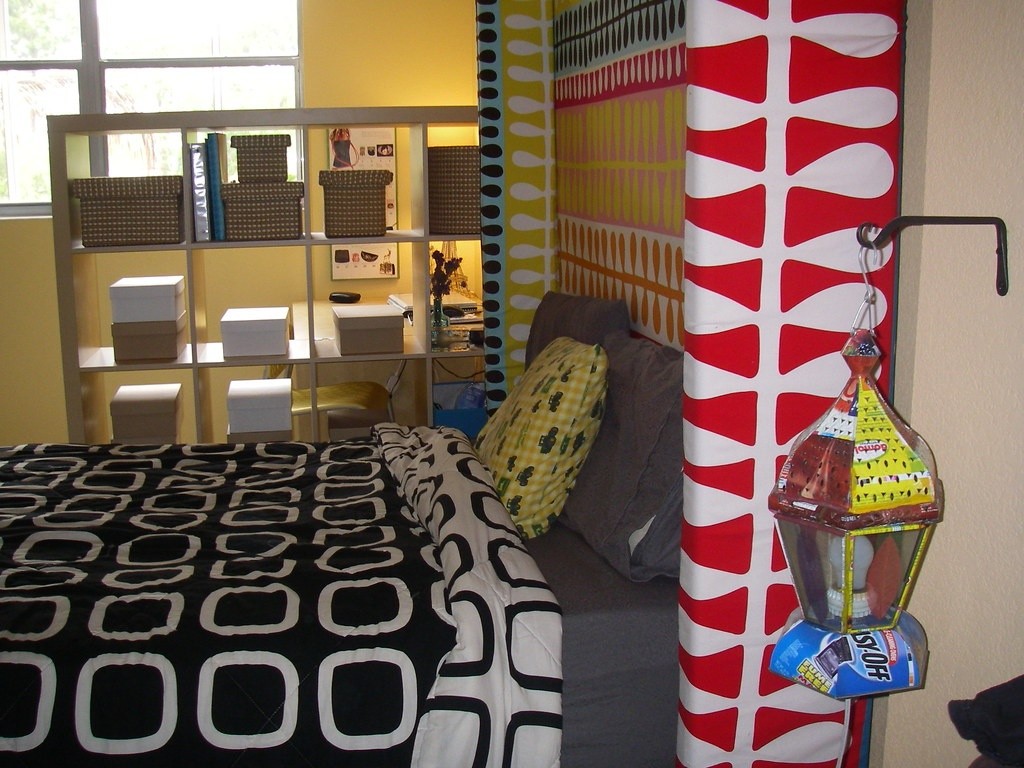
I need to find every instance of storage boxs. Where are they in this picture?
[71,175,186,247]
[221,182,304,242]
[108,275,185,323]
[111,311,187,362]
[227,379,293,433]
[110,382,184,438]
[327,408,390,442]
[220,307,291,357]
[318,169,393,238]
[332,305,404,356]
[226,425,294,444]
[230,134,292,183]
[433,381,488,440]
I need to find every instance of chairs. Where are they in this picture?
[291,359,408,423]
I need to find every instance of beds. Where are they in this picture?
[0,0,908,768]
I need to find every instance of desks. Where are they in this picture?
[291,297,484,442]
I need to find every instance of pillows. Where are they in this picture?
[525,290,631,372]
[473,336,610,541]
[554,333,684,584]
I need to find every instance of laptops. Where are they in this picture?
[387,292,478,317]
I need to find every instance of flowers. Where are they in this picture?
[428,249,463,299]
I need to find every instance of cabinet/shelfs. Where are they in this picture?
[45,105,479,445]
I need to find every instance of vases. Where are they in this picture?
[431,299,450,352]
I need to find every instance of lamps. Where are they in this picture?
[769,215,1009,702]
